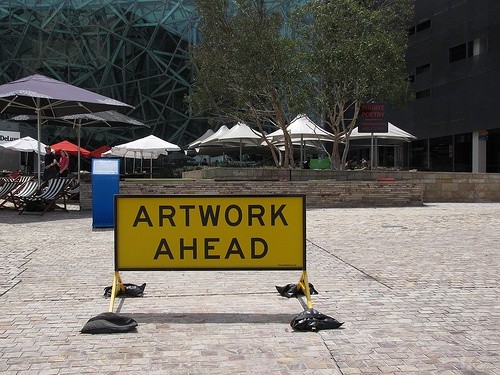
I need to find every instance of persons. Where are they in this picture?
[41,147,58,181]
[58,151,69,178]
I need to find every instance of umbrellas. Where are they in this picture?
[267,113,336,165]
[190,123,267,166]
[339,122,417,170]
[50,141,90,156]
[0,136,48,171]
[91,145,111,157]
[102,135,181,177]
[1,74,150,190]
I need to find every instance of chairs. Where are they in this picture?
[0,169,80,216]
